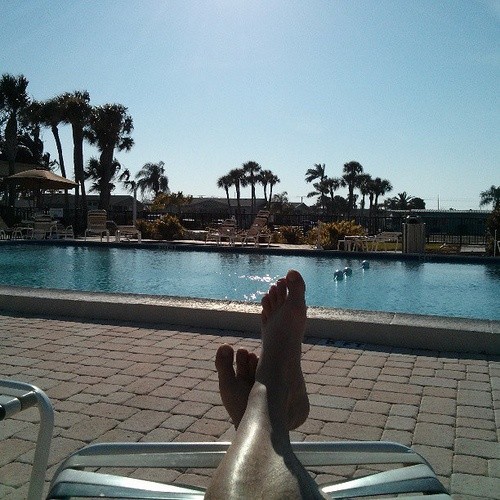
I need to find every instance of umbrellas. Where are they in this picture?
[4,166,79,212]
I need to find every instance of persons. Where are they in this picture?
[203,269,316,499]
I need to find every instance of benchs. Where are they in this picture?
[0,206,402,255]
[0,378,54,500]
[43,439,451,500]
